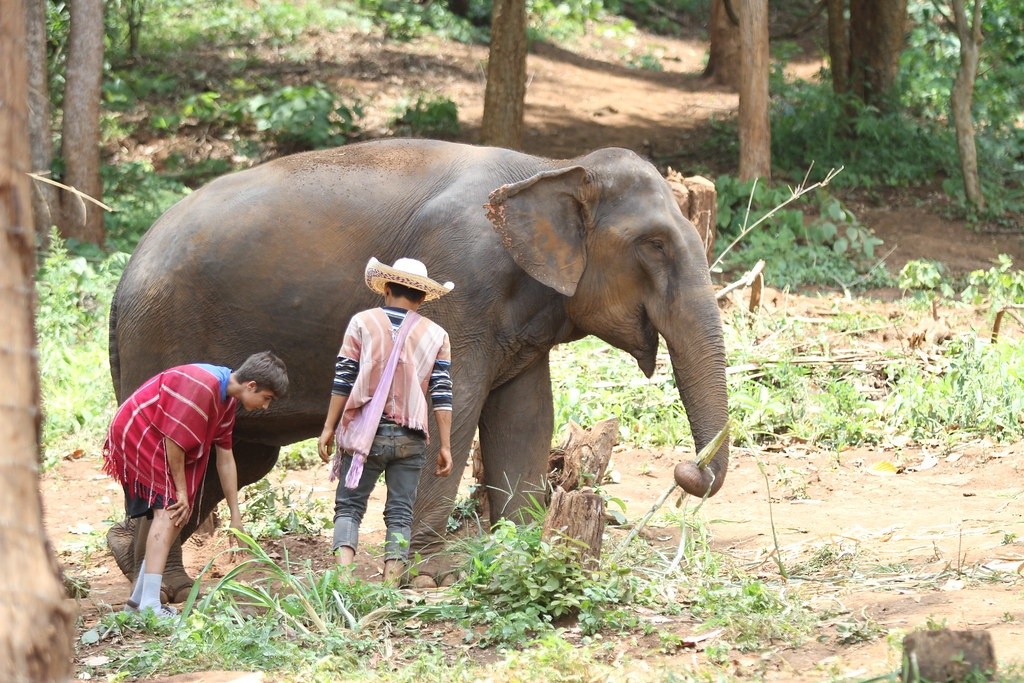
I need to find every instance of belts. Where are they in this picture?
[377,426,420,437]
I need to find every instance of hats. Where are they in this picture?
[364,257,455,301]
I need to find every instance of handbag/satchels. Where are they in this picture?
[329,399,385,488]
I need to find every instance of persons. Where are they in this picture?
[102,349,290,627]
[317,256,454,589]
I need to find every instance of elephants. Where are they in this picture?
[104,137,730,607]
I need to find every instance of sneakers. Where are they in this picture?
[124,600,187,627]
